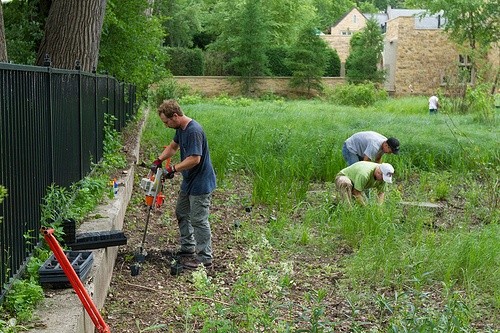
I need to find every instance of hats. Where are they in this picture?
[386,137,400,155]
[380,163,394,184]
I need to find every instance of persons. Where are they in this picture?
[342,130,401,198]
[334,161,394,211]
[428,90,442,114]
[151,100,216,270]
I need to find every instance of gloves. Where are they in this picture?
[164,165,177,179]
[151,158,163,174]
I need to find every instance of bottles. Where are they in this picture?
[113,179,118,196]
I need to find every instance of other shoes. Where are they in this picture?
[183,260,213,270]
[178,250,195,256]
